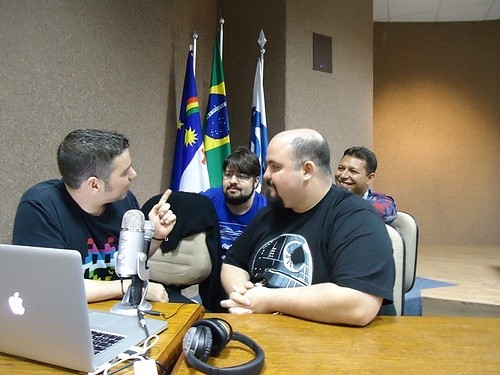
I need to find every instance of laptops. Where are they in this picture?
[0,244,168,372]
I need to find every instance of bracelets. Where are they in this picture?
[152,236,169,241]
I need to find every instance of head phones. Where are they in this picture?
[182,317,265,375]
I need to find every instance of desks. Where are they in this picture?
[0,298,500,375]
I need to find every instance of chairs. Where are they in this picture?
[378,210,419,315]
[139,190,221,306]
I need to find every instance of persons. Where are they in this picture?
[221,128,397,327]
[12,130,196,303]
[334,146,396,225]
[199,146,269,259]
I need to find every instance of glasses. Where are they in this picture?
[220,169,254,182]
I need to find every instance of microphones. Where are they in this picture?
[116,209,154,305]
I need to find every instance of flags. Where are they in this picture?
[173,46,209,194]
[205,30,231,187]
[249,59,268,195]
